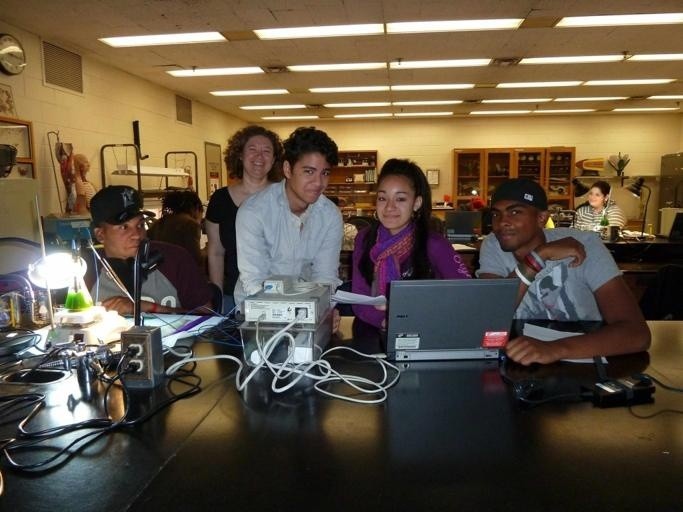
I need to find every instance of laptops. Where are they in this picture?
[385,278,520,360]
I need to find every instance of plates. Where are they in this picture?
[0,331,34,356]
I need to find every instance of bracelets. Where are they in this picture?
[513,252,545,286]
[152,302,159,314]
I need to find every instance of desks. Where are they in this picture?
[601,236,683,274]
[0,316,683,512]
[432,204,453,219]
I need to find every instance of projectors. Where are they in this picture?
[240,302,341,367]
[245,283,331,324]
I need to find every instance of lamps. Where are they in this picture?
[28,237,105,325]
[624,176,651,239]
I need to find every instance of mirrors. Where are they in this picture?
[205,142,222,200]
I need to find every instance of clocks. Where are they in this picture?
[0,34,25,74]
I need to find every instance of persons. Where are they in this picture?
[72,151,97,196]
[206,126,285,314]
[54,186,214,315]
[140,188,223,314]
[570,180,625,241]
[478,176,652,366]
[352,158,472,330]
[235,127,346,335]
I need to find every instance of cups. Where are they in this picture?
[32,290,56,324]
[357,208,362,216]
[608,225,621,241]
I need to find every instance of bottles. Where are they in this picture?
[648,224,652,238]
[65,235,93,311]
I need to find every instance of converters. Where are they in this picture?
[264,274,293,294]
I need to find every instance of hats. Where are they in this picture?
[491,179,548,211]
[90,186,155,225]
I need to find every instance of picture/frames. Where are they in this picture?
[426,170,439,185]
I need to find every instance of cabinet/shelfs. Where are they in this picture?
[324,151,378,217]
[452,149,514,211]
[101,145,198,209]
[514,147,576,210]
[0,116,37,179]
